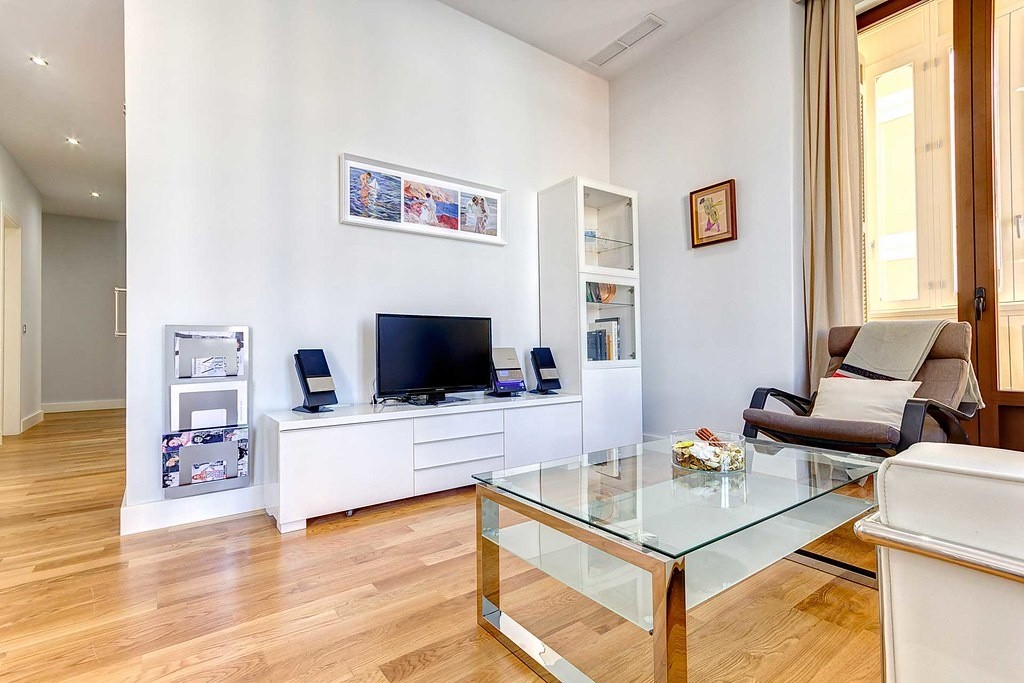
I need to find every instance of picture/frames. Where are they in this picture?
[339,153,508,245]
[690,179,737,248]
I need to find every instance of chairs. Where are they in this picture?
[743,320,986,463]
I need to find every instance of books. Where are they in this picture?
[587,316,622,361]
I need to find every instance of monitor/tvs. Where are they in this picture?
[375,313,493,406]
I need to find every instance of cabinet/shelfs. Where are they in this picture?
[261,393,583,534]
[537,175,643,467]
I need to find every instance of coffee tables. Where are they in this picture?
[471,434,887,683]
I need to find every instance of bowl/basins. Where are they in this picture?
[672,429,745,472]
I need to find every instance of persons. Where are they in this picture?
[360,173,371,189]
[420,193,438,222]
[161,426,249,488]
[471,196,489,235]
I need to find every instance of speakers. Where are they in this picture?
[529,347,561,395]
[292,349,338,413]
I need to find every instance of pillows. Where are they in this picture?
[810,377,923,430]
